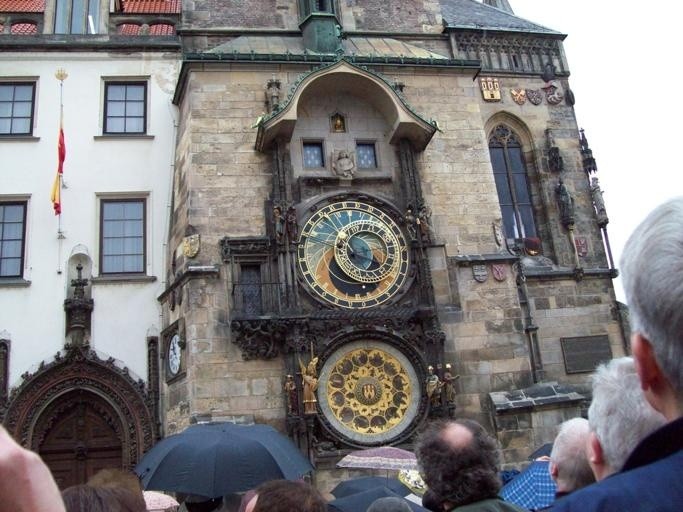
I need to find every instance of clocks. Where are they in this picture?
[291,190,422,315]
[166,332,182,376]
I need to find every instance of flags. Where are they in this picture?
[52,128,66,216]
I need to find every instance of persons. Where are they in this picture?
[286,206,299,245]
[443,362,460,404]
[549,195,683,512]
[284,374,298,417]
[333,150,355,177]
[294,353,319,414]
[586,356,669,482]
[422,365,446,409]
[405,206,434,240]
[271,208,285,244]
